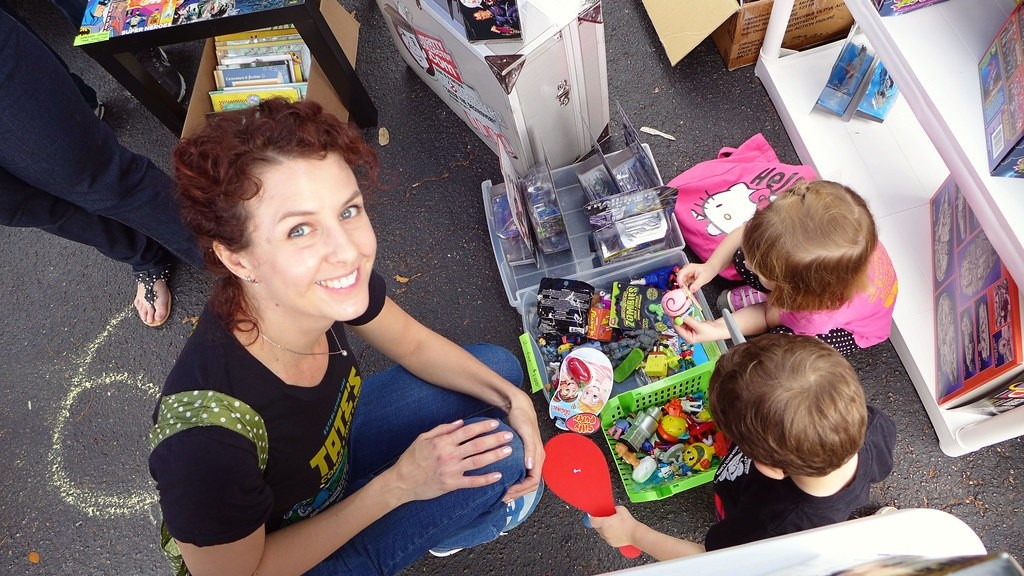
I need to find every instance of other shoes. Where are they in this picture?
[93,99,105,120]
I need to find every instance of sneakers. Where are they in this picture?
[428,475,545,557]
[717,283,770,315]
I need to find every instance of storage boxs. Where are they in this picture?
[976,2,1024,182]
[811,25,897,124]
[180,1,360,139]
[479,143,740,502]
[641,1,855,71]
[929,174,1022,418]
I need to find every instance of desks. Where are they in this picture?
[73,1,378,140]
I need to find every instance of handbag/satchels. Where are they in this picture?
[664,133,815,282]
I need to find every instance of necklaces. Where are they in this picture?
[242,315,348,357]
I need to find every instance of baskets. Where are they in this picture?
[599,361,715,502]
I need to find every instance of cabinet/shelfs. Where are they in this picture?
[754,1,1023,456]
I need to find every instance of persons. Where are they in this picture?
[671,180,899,359]
[150,95,546,576]
[0,3,226,326]
[588,331,897,562]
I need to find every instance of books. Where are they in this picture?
[456,0,524,44]
[205,23,312,125]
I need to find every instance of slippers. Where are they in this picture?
[134,271,171,327]
